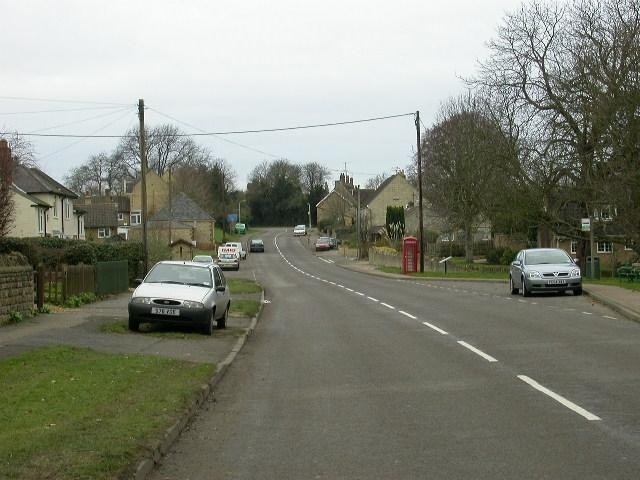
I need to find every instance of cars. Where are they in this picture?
[507,246,583,297]
[249,239,264,252]
[217,252,239,270]
[316,235,337,250]
[235,223,246,234]
[292,224,307,236]
[188,254,214,264]
[126,260,234,334]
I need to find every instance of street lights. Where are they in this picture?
[306,201,313,231]
[239,198,247,222]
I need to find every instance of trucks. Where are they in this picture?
[218,240,248,260]
